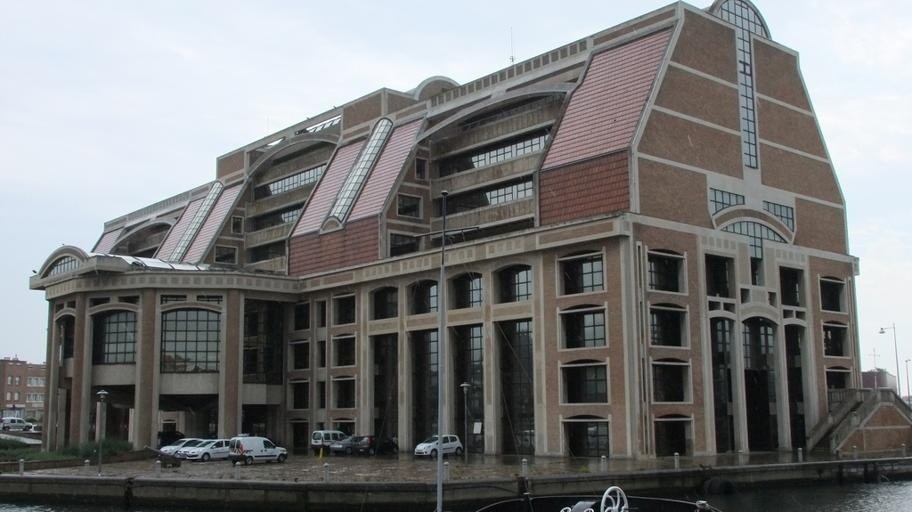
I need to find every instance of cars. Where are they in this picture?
[160,438,232,462]
[415,434,464,458]
[329,436,363,456]
[0,417,32,431]
[353,436,398,456]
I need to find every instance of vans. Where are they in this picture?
[311,430,347,456]
[229,436,288,465]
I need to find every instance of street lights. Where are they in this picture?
[98,390,108,473]
[460,382,472,464]
